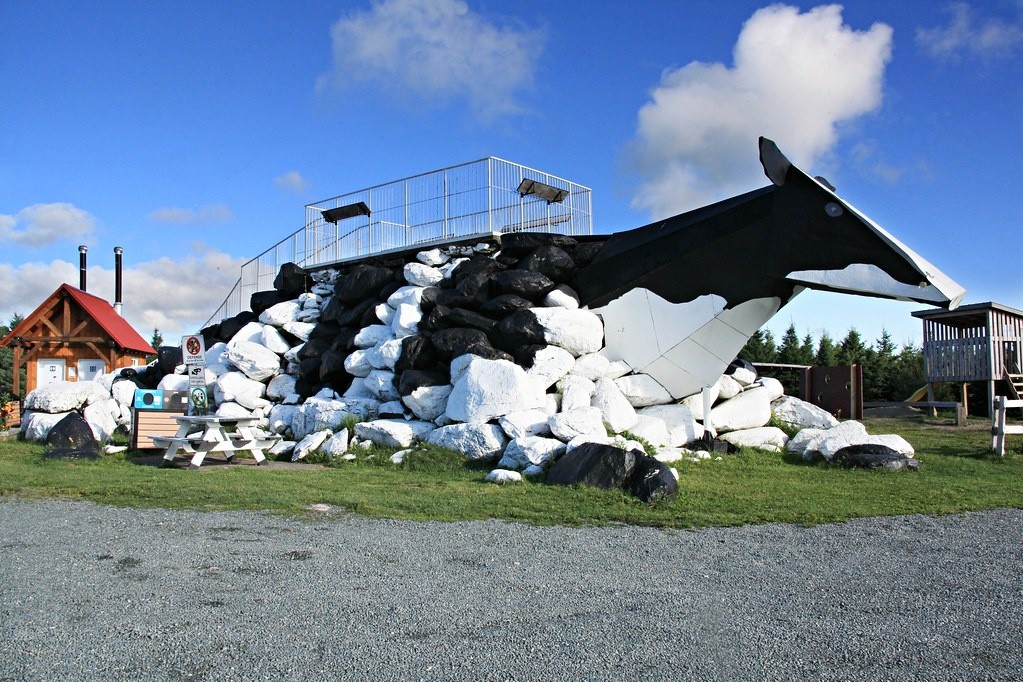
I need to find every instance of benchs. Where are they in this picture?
[228,432,285,439]
[147,435,221,445]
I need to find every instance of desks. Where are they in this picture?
[157,415,268,468]
